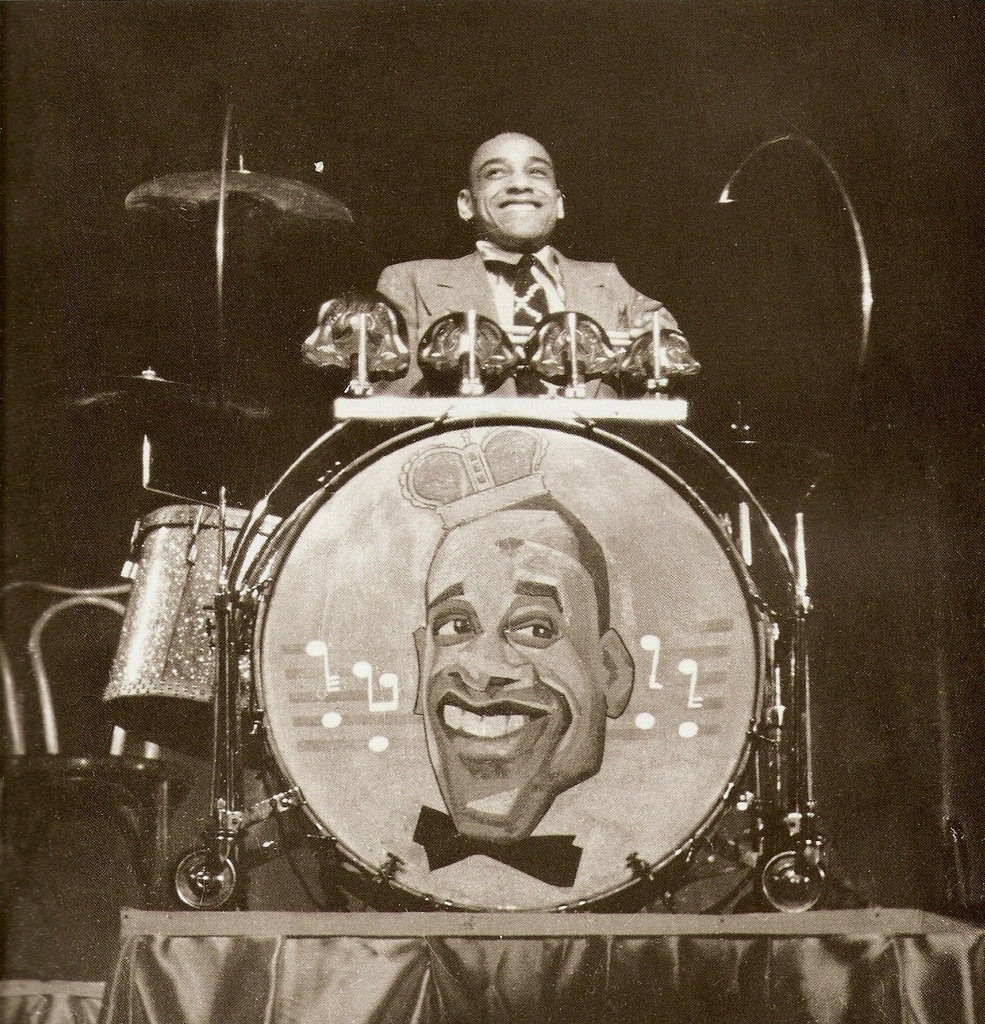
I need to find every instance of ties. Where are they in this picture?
[511,254,552,398]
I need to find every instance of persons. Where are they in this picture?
[367,131,683,400]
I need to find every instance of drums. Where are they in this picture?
[242,413,766,918]
[101,504,289,770]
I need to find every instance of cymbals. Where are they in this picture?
[121,171,355,224]
[34,373,274,419]
